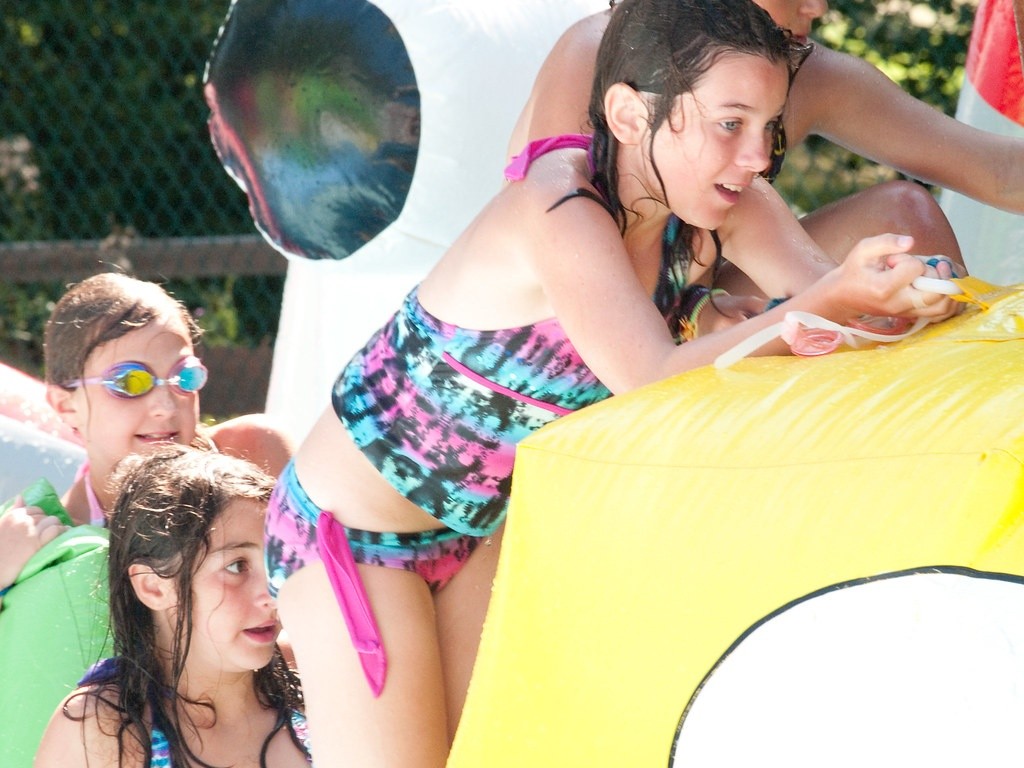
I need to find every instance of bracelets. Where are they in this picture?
[671,284,730,343]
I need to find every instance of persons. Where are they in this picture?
[1,360,82,447]
[0,269,307,717]
[508,1,1024,335]
[265,0,960,768]
[34,439,313,768]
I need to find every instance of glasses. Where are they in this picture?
[786,310,916,358]
[60,353,210,400]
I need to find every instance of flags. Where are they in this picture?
[937,6,1024,282]
